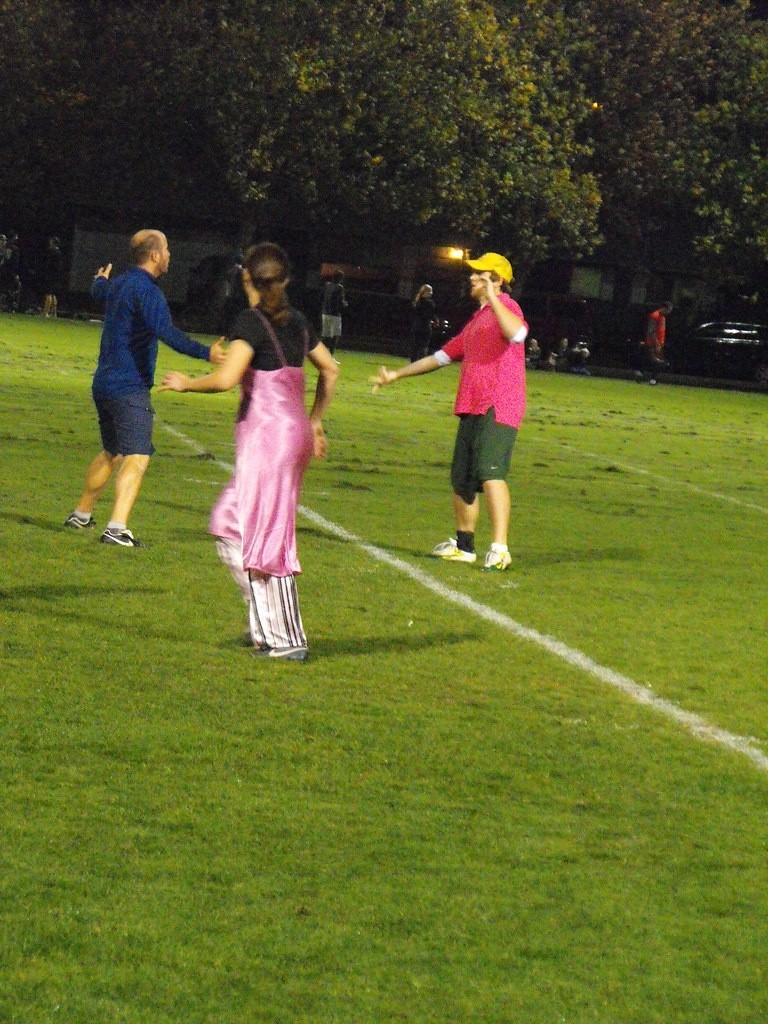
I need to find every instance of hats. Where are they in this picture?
[465,252,513,284]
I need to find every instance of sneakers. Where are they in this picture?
[481,551,512,572]
[431,537,477,563]
[100,530,146,547]
[64,514,97,529]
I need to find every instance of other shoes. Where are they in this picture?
[238,631,253,647]
[634,369,644,384]
[252,646,307,660]
[333,357,341,365]
[649,379,657,386]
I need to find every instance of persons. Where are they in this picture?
[525,338,591,376]
[0,230,22,314]
[318,270,348,365]
[368,253,528,572]
[411,285,440,363]
[223,253,244,333]
[38,237,63,317]
[64,230,231,547]
[634,302,672,385]
[156,243,341,659]
